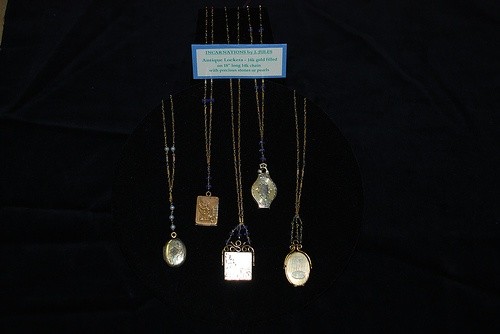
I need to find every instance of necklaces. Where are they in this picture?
[283,89,313,287]
[221,6,255,281]
[162,95,187,268]
[246,4,278,209]
[195,7,219,227]
